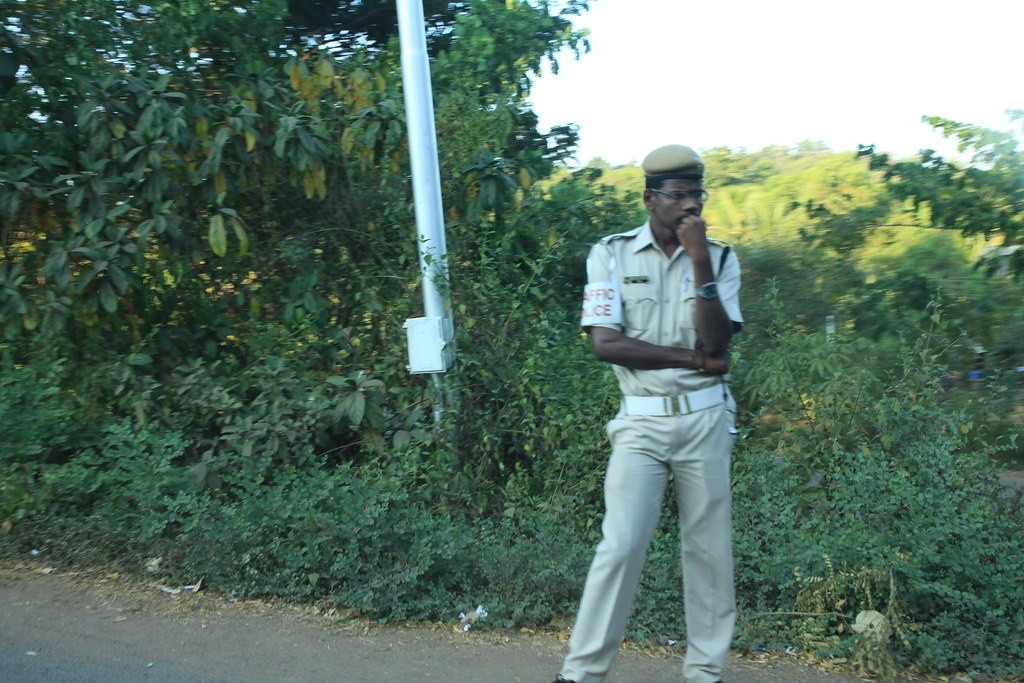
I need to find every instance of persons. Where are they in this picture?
[553,145,745,683]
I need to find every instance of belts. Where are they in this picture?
[622,383,730,416]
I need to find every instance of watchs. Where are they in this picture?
[696,282,718,299]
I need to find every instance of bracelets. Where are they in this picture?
[698,351,705,373]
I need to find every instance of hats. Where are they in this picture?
[642,144,705,180]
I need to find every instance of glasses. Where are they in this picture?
[650,188,710,204]
